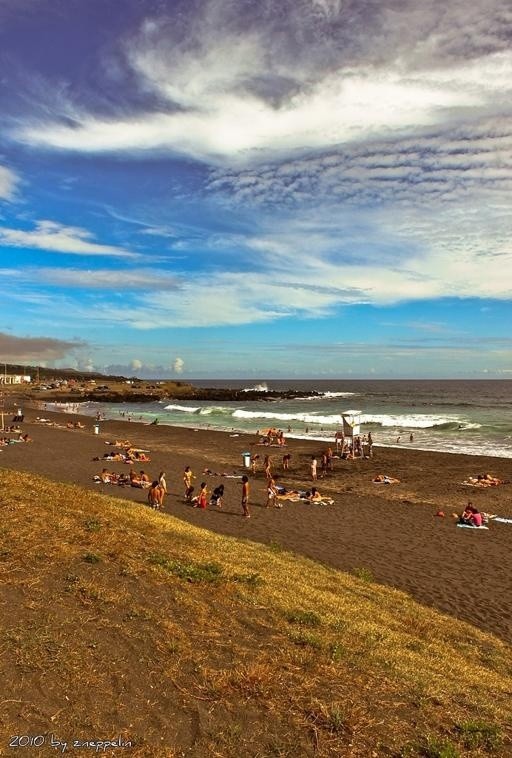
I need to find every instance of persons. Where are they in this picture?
[397,437,401,443]
[375,475,394,482]
[410,434,413,441]
[44,403,47,412]
[237,425,373,516]
[54,397,143,429]
[92,439,225,510]
[460,474,510,527]
[0,425,33,446]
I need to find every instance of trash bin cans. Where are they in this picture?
[241,452,251,468]
[93,424,99,435]
